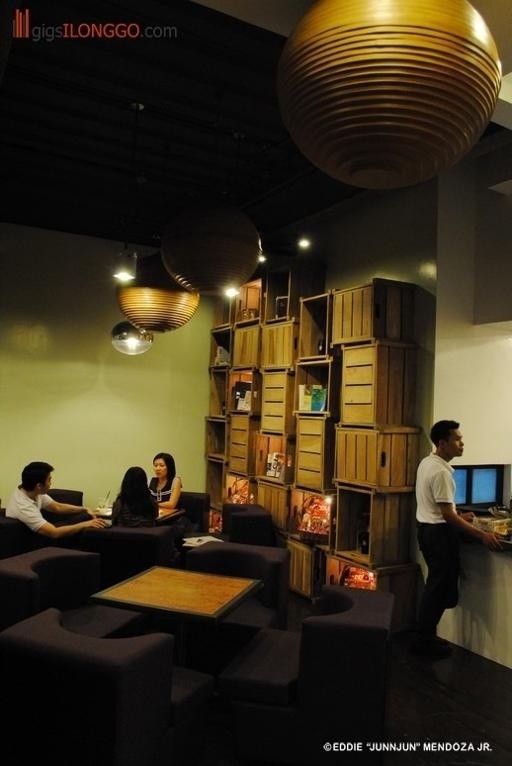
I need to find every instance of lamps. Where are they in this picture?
[110,208,317,357]
[277,0,503,192]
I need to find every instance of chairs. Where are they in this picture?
[1,488,396,765]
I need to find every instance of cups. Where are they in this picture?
[97,496,109,513]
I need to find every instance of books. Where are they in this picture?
[298,384,327,412]
[266,451,292,479]
[232,380,252,409]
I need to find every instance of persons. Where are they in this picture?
[409,420,506,659]
[111,466,159,528]
[5,461,109,539]
[148,452,187,553]
[278,301,285,317]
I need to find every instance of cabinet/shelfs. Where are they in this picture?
[204,264,436,633]
